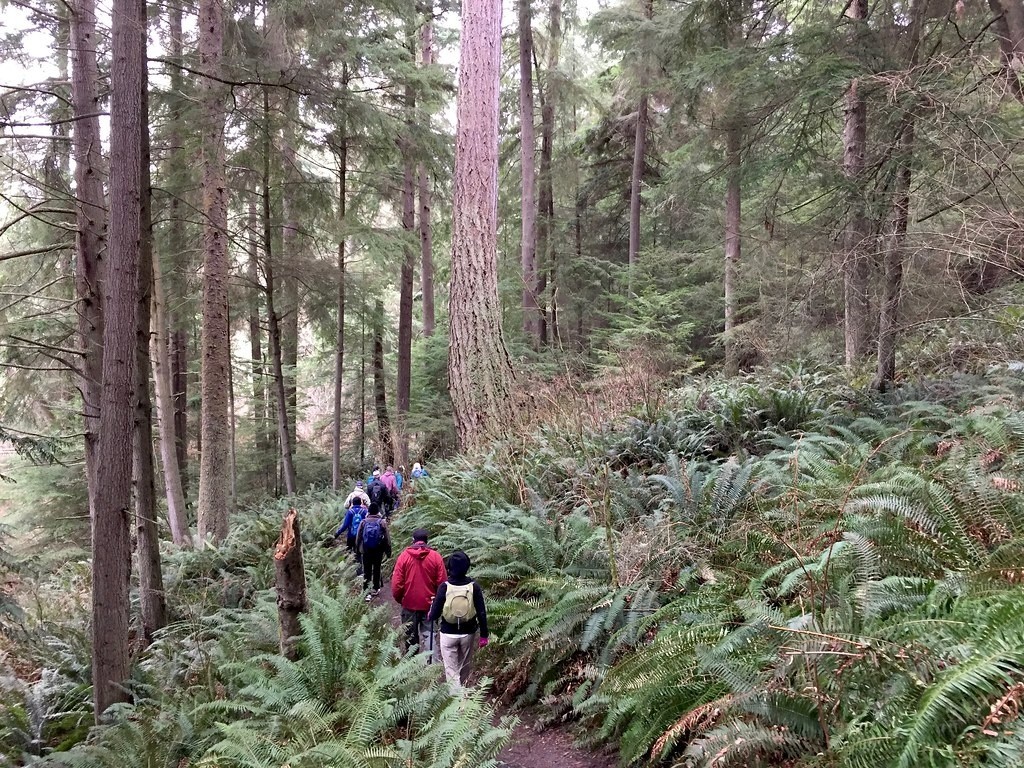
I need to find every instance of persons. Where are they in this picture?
[356,502,392,589]
[392,530,448,657]
[430,551,489,693]
[335,497,366,575]
[336,462,424,511]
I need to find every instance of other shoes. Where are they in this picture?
[371,589,381,595]
[365,594,371,601]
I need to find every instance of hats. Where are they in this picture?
[369,502,378,512]
[372,465,379,472]
[447,551,470,577]
[355,481,363,486]
[387,466,392,471]
[353,497,360,505]
[413,529,428,541]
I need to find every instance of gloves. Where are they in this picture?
[427,611,431,621]
[478,637,489,648]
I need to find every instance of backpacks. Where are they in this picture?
[350,491,365,506]
[349,508,365,536]
[362,518,382,550]
[414,469,421,478]
[370,482,382,502]
[441,580,477,624]
